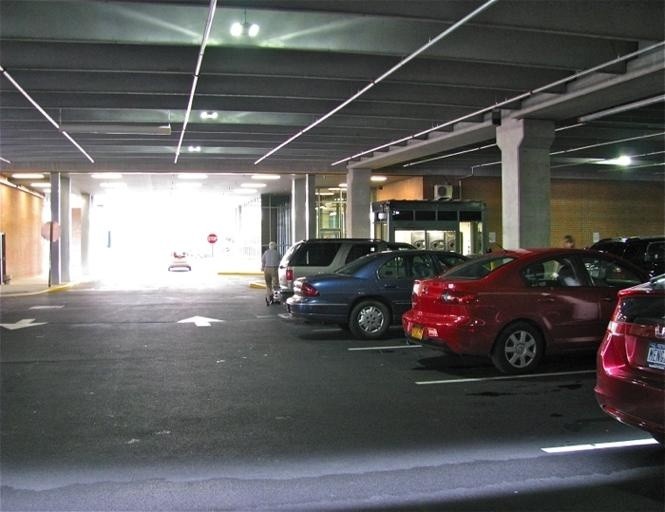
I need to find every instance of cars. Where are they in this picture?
[167,249,191,271]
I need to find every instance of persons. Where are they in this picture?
[560,235,575,249]
[261,241,283,302]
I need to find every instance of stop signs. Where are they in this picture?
[207,233,218,244]
